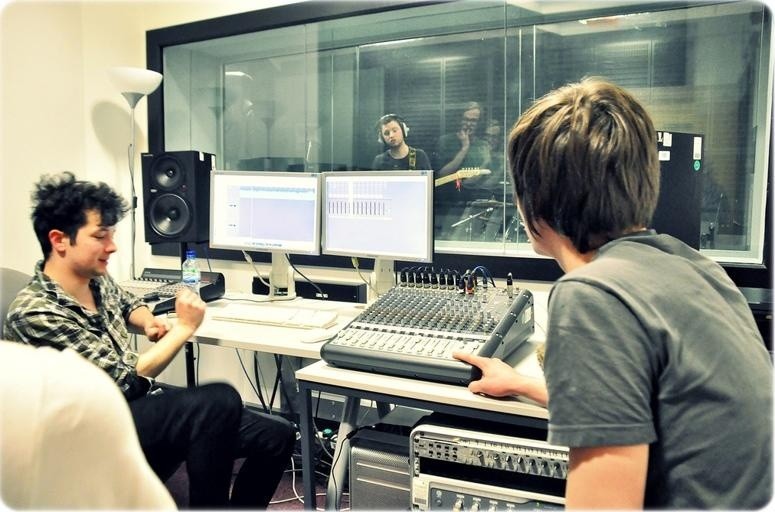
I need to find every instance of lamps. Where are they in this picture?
[107,65,163,353]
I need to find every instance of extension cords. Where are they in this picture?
[317,432,341,449]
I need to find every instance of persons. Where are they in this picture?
[1,172,298,509]
[436,98,491,238]
[475,117,512,242]
[449,75,774,510]
[370,111,433,171]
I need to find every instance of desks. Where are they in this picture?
[294,334,553,509]
[130,289,392,510]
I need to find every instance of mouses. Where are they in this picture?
[302,325,330,343]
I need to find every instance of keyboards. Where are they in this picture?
[211,302,337,330]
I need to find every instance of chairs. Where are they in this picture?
[0,268,31,336]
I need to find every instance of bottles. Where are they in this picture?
[182,251,200,297]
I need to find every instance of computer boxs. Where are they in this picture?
[348,406,430,511]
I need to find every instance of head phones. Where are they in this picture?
[376,113,410,144]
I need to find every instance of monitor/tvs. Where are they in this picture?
[321,170,435,306]
[208,170,318,303]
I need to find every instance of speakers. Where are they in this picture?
[141,151,215,245]
[651,128,705,252]
